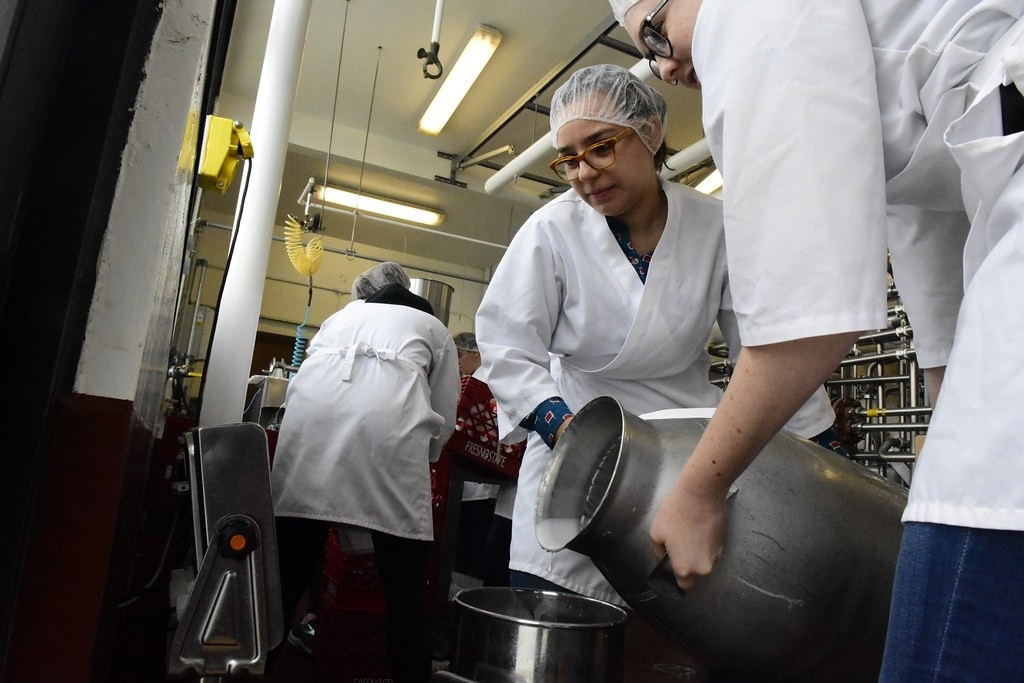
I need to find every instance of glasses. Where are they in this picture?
[639,0,679,85]
[549,126,635,182]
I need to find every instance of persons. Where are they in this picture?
[270,262,500,683]
[606,0,1024,683]
[472,64,837,610]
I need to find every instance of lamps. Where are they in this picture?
[416,23,502,138]
[311,182,446,227]
[689,167,724,194]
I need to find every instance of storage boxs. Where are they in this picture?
[312,570,434,683]
[323,448,452,592]
[440,374,527,486]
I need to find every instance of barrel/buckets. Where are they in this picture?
[542,394,910,683]
[407,277,455,329]
[434,586,627,683]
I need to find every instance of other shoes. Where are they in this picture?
[285,616,324,654]
